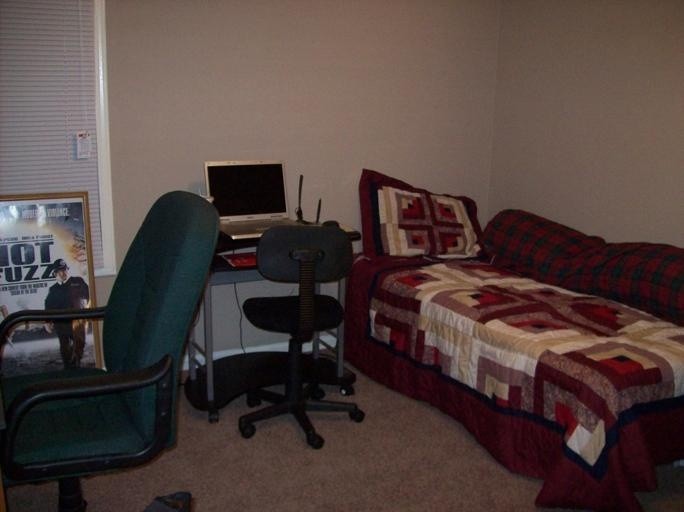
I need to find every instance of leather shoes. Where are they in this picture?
[74,356,80,368]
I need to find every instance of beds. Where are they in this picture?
[346,252,684,512]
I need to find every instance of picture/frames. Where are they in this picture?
[0,191,103,377]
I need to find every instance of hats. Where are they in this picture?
[51,259,69,274]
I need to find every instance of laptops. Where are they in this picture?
[203,159,304,240]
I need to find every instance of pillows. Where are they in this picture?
[359,168,485,269]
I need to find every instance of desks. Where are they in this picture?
[185,225,361,424]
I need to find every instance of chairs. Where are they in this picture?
[239,226,365,449]
[0,190,219,512]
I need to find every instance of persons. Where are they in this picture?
[44,258,90,367]
